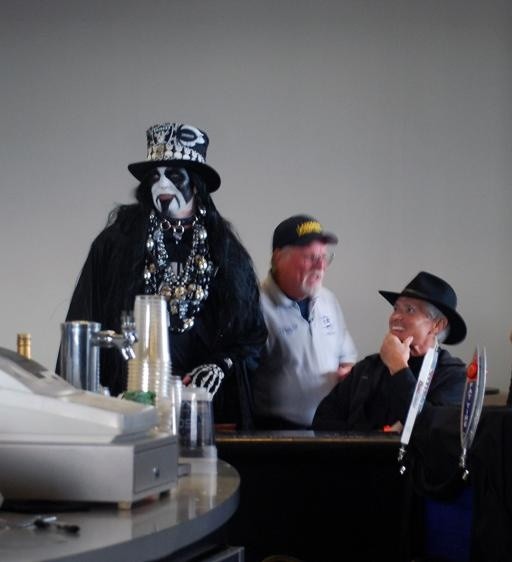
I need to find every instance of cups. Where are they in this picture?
[126,293,218,475]
[178,475,218,525]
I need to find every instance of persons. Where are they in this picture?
[247,214,359,425]
[55,118,272,440]
[310,268,468,452]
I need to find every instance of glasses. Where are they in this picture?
[305,252,335,268]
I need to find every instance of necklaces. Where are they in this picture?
[143,209,215,337]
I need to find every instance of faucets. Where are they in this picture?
[94,321,137,364]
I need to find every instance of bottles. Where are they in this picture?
[16,333,31,358]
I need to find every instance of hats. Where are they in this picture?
[127,120,223,196]
[272,213,340,251]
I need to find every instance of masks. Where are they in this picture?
[377,270,469,347]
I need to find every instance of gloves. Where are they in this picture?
[184,356,236,401]
